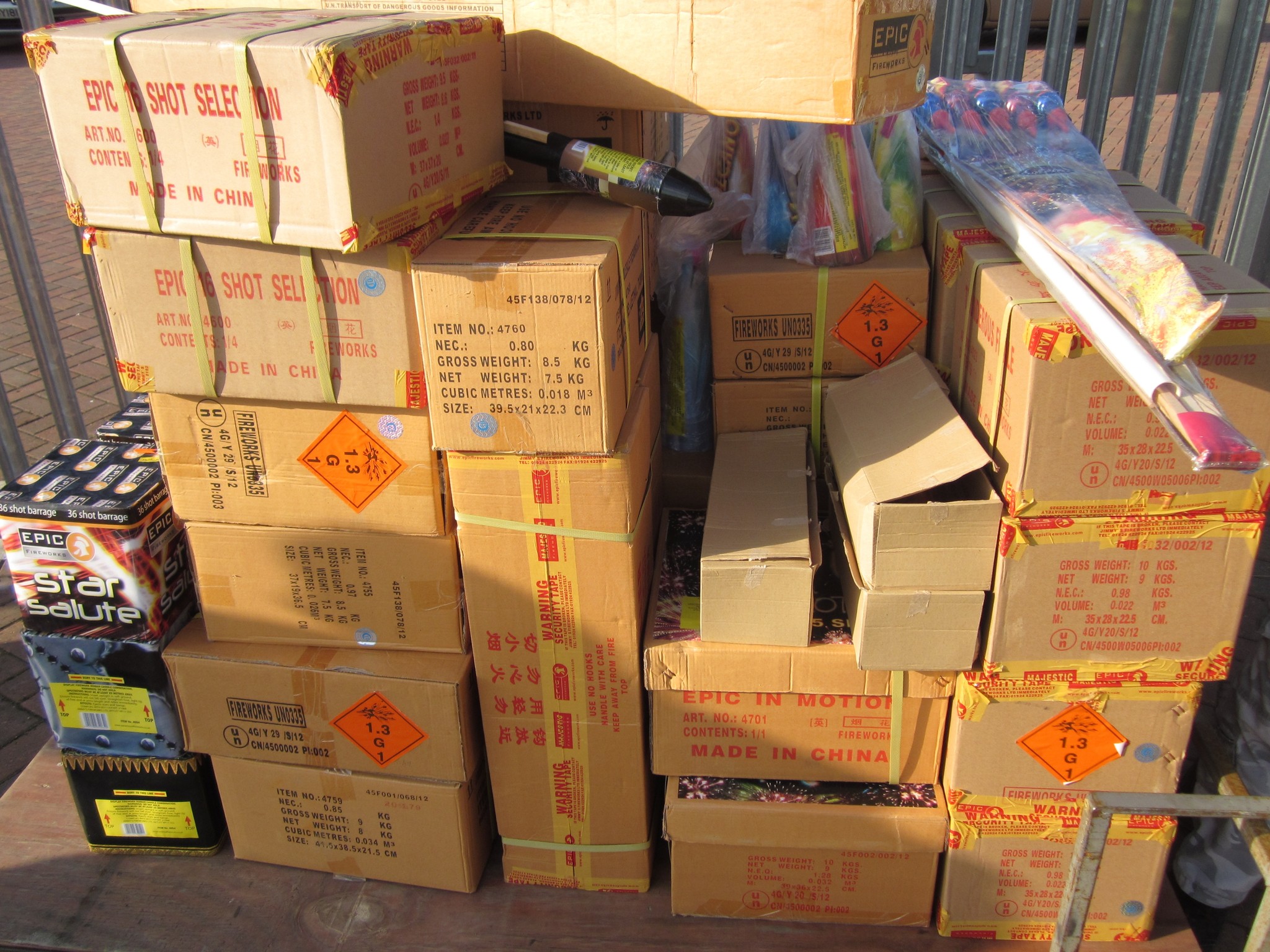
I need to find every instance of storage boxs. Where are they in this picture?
[0,0,1270,944]
